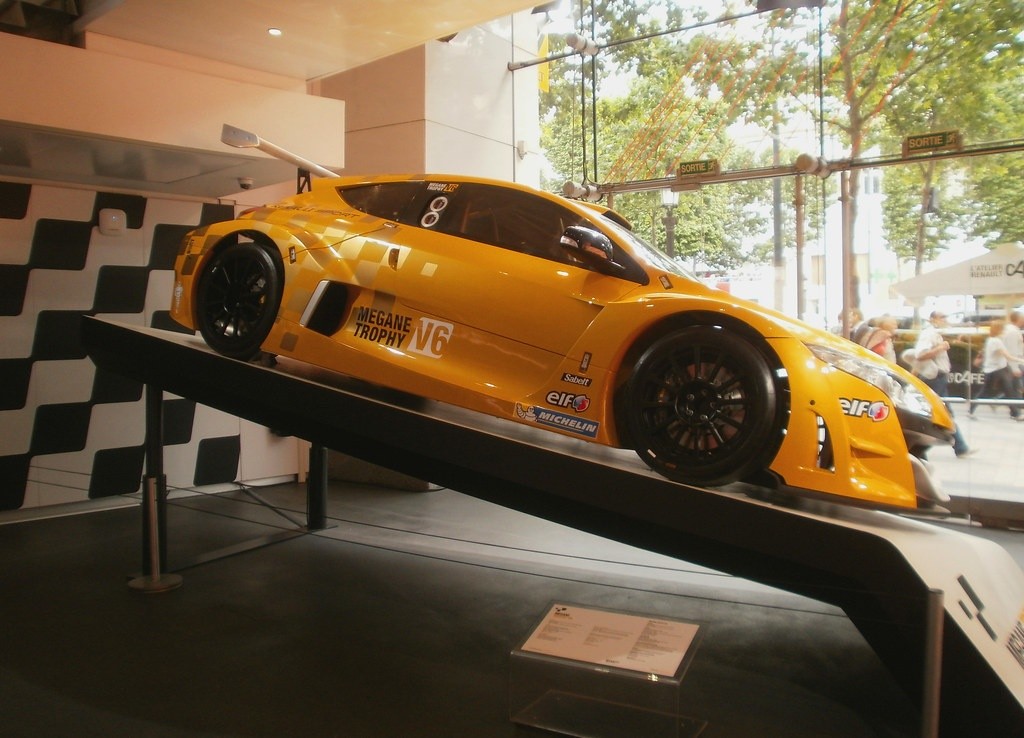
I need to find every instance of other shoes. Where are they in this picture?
[1010,413,1024,420]
[968,399,978,414]
[955,447,977,458]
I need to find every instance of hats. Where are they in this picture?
[930,311,948,319]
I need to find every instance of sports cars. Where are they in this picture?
[168,121,971,520]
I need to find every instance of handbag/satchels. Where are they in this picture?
[900,349,939,379]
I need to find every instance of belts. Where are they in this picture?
[938,372,949,376]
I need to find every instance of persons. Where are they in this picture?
[967,312,1024,421]
[901,310,977,462]
[848,309,897,366]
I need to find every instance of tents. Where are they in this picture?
[888,241,1024,329]
